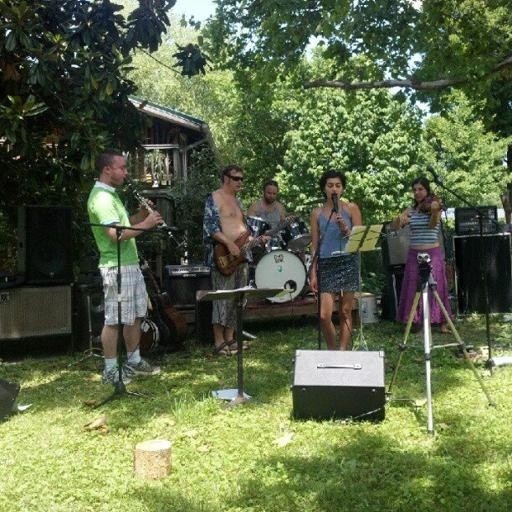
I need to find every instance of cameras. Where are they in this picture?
[417,251,431,262]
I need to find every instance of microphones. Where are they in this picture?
[330,190,341,214]
[157,224,180,235]
[427,164,442,183]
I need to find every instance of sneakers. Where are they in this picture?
[123,359,162,375]
[101,365,132,385]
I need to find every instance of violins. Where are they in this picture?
[418,192,449,215]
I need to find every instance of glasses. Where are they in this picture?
[224,174,244,182]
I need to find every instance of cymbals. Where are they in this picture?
[302,197,327,207]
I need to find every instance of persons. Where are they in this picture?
[245,179,288,231]
[308,168,364,351]
[86,148,162,387]
[388,175,453,335]
[201,163,274,356]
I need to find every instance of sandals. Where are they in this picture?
[213,342,238,355]
[229,339,249,349]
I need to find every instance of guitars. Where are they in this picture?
[213,214,297,277]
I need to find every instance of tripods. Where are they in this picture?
[386,270,497,440]
[351,251,368,350]
[90,229,148,410]
[306,208,335,350]
[210,296,266,412]
[455,214,512,368]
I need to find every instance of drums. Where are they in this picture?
[277,220,313,251]
[253,248,309,305]
[242,215,271,241]
